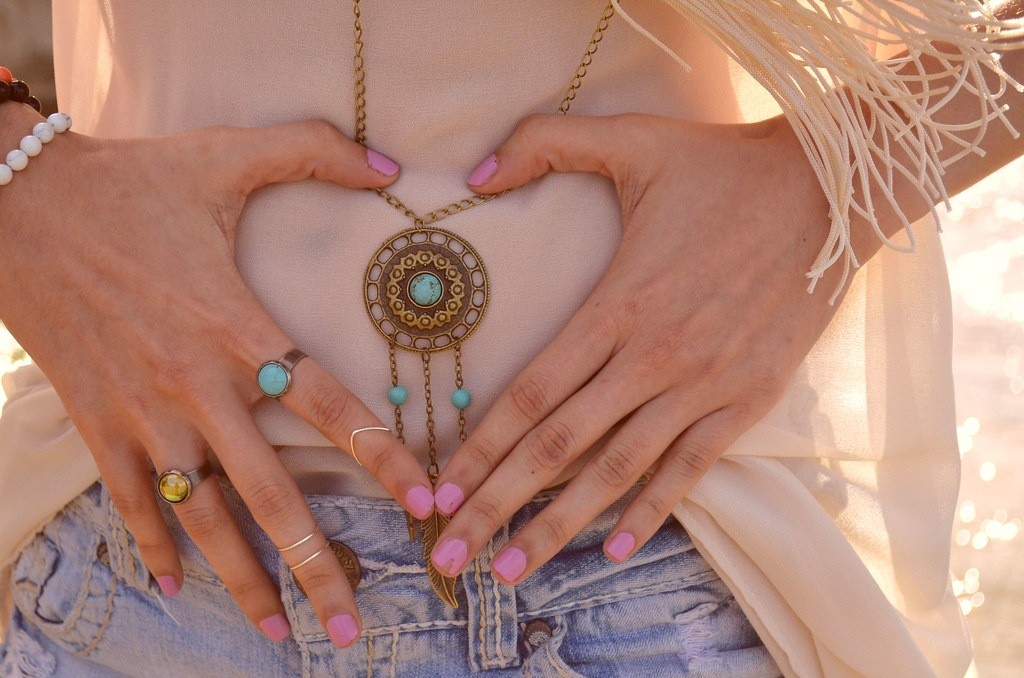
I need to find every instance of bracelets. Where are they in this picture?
[0,64,74,189]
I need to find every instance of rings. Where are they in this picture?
[155,460,216,507]
[350,426,393,471]
[286,540,332,573]
[254,349,309,398]
[274,522,319,555]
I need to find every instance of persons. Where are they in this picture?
[2,5,1024,678]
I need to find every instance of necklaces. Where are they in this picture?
[343,0,622,613]
